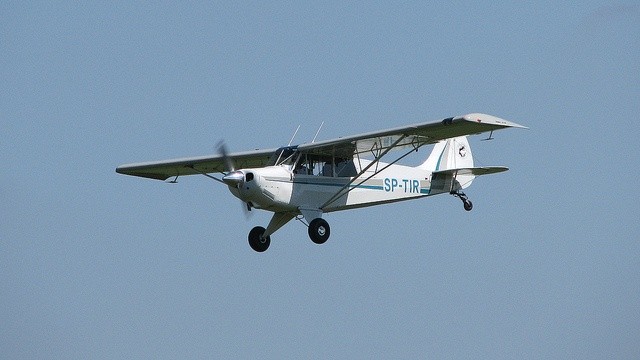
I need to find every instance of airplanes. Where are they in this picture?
[116,111,528,252]
